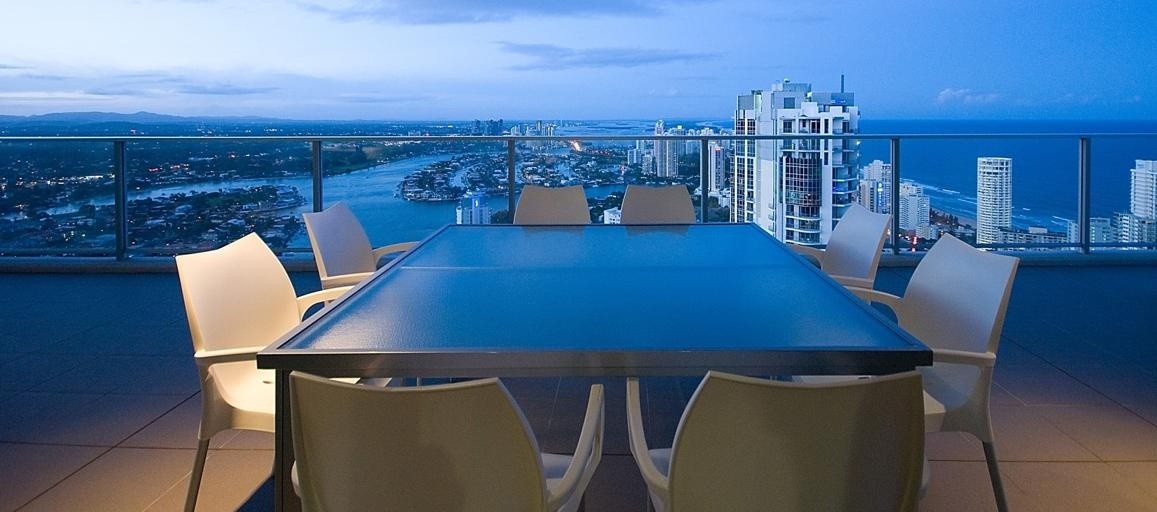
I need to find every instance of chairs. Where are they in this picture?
[512,185,591,225]
[175,231,394,512]
[784,204,892,306]
[302,201,422,308]
[625,370,925,512]
[288,370,605,512]
[843,233,1022,512]
[620,185,696,225]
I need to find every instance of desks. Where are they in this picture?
[255,222,933,511]
